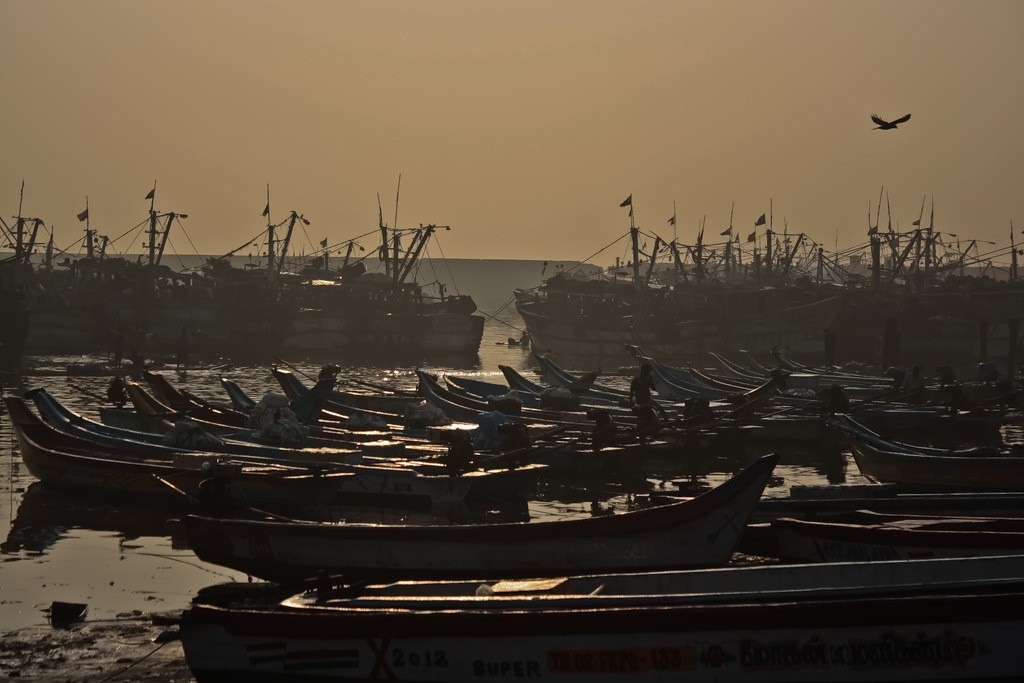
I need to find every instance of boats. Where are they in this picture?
[1,177,485,365]
[2,344,1023,682]
[508,192,1024,363]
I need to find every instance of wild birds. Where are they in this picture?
[870,113,912,130]
